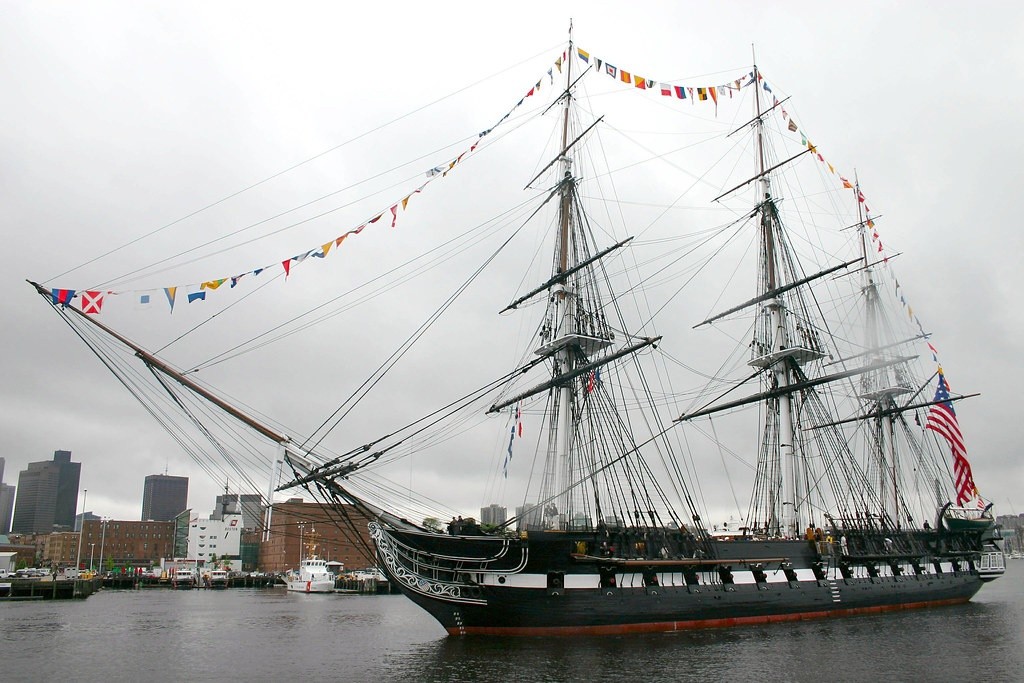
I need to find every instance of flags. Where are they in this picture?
[758,64,960,375]
[575,47,755,113]
[50,36,571,320]
[926,367,987,508]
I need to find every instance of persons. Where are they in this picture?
[450,516,464,534]
[815,530,849,556]
[543,503,560,531]
[923,520,932,531]
[52,571,57,581]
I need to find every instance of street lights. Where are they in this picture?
[99,515,110,575]
[297,521,306,569]
[89,543,95,571]
[75,488,87,577]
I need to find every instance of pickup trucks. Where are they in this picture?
[0,568,16,579]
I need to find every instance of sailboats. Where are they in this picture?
[27,17,1011,640]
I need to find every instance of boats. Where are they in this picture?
[943,515,994,530]
[172,569,195,589]
[206,569,230,589]
[287,519,336,592]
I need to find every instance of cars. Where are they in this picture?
[15,567,51,577]
[278,572,286,578]
